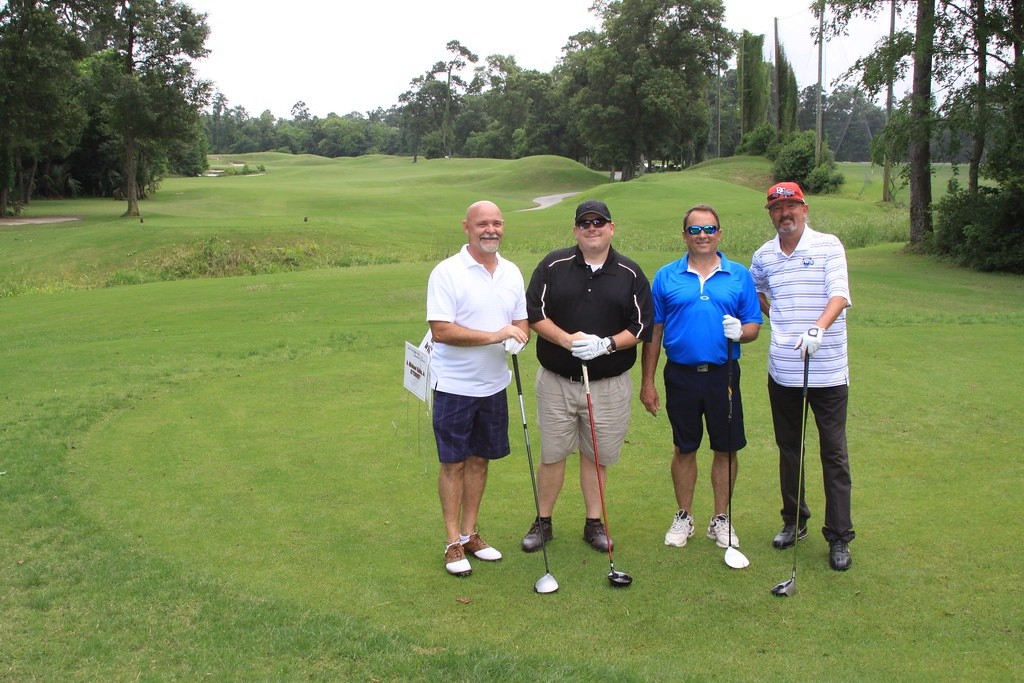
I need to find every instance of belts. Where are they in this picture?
[560,372,598,383]
[697,364,714,372]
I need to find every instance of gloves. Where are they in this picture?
[722,315,743,342]
[793,325,824,358]
[502,337,525,355]
[571,334,611,360]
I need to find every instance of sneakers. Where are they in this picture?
[829,539,852,570]
[773,522,807,549]
[444,542,472,576]
[462,525,502,560]
[665,509,696,547]
[706,513,739,548]
[523,518,552,552]
[584,522,613,551]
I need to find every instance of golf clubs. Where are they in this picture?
[724,338,752,569]
[511,353,561,596]
[770,347,811,598]
[581,362,635,585]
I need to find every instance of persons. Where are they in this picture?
[523,201,654,554]
[640,206,764,548]
[426,201,530,577]
[748,182,852,570]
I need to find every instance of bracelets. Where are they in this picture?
[608,336,616,353]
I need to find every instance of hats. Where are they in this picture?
[765,182,806,209]
[575,200,612,224]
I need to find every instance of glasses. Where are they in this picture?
[686,225,717,235]
[577,218,610,229]
[767,190,795,201]
